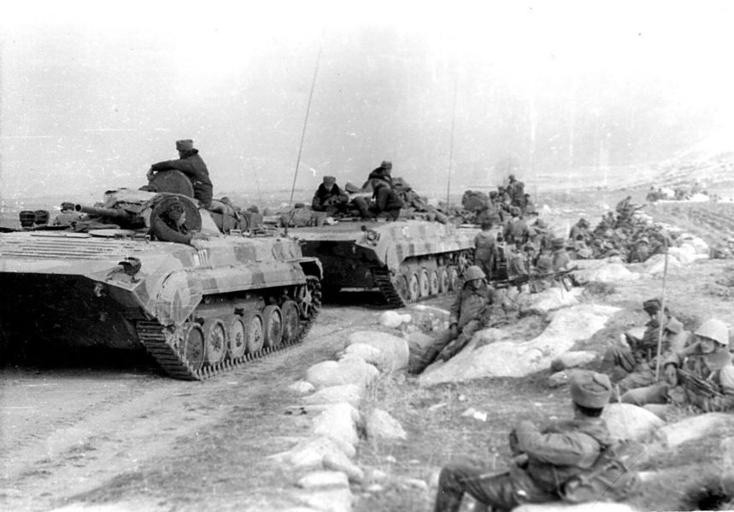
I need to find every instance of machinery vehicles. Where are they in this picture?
[0,49,324,381]
[262,63,502,309]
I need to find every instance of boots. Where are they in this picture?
[409,347,439,373]
[440,337,468,361]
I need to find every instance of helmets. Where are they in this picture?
[694,317,730,347]
[464,266,486,281]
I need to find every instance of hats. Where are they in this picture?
[176,139,194,152]
[324,176,336,185]
[568,369,614,408]
[644,297,668,315]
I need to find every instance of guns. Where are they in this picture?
[677,367,726,399]
[495,265,578,293]
[459,454,529,482]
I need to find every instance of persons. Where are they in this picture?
[19,137,267,237]
[405,175,590,374]
[431,370,612,511]
[608,319,733,413]
[567,194,669,265]
[280,160,431,224]
